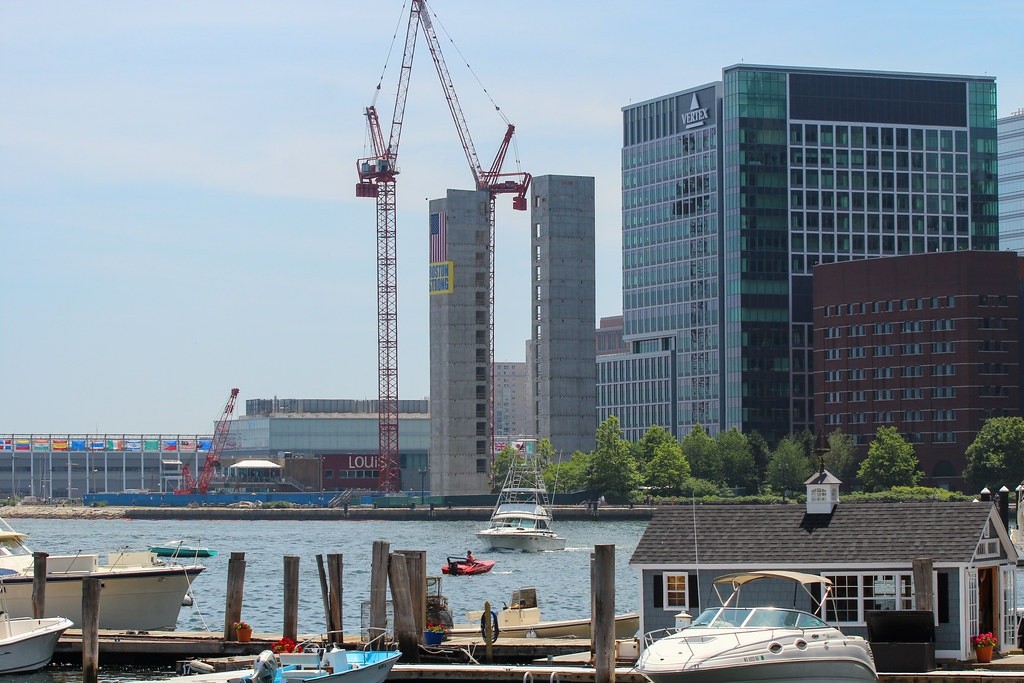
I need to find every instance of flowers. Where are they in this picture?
[232,621,253,630]
[970,632,997,652]
[423,623,448,634]
[271,639,300,655]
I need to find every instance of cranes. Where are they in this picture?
[355,0,533,496]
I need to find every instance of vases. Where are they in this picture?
[424,632,444,647]
[236,628,252,642]
[976,646,993,663]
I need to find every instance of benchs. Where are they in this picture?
[280,653,326,678]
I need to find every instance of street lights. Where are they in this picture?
[418,464,427,505]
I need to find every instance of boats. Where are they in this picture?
[441,556,496,575]
[146,540,219,558]
[473,439,566,551]
[633,491,878,683]
[1010,485,1024,566]
[167,627,403,683]
[0,516,207,674]
[446,587,640,639]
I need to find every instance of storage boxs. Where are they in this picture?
[864,609,936,672]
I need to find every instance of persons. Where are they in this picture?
[467,550,473,566]
[598,494,604,504]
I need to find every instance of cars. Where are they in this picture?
[208,501,256,508]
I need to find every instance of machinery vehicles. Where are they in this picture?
[172,387,241,495]
